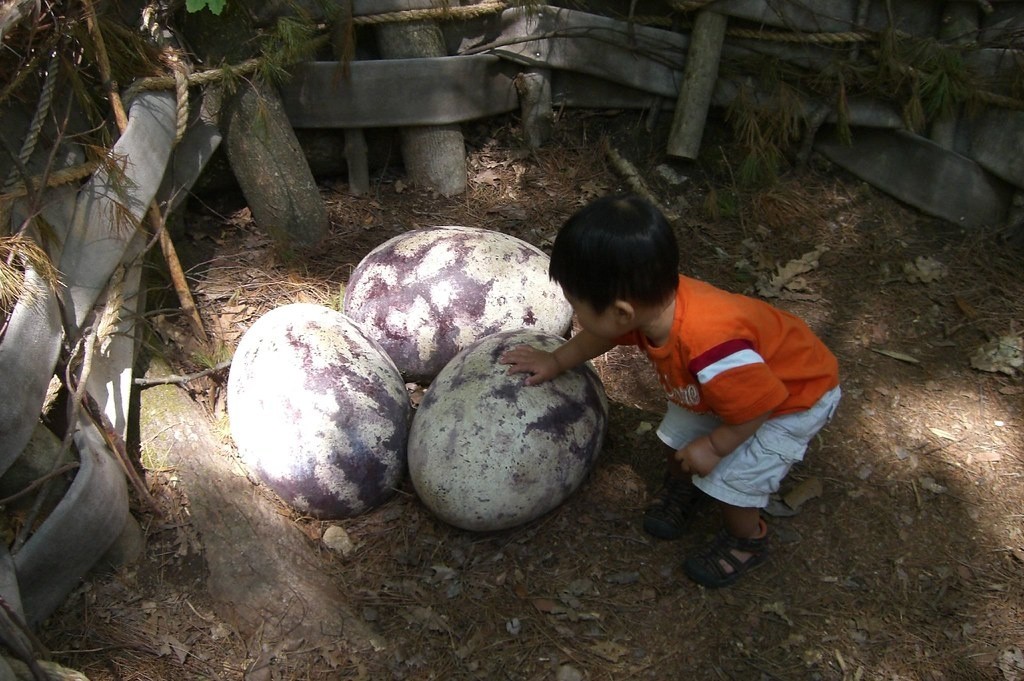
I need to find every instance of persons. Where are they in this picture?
[498,190,843,589]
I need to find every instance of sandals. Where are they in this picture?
[643,469,706,540]
[684,518,770,589]
